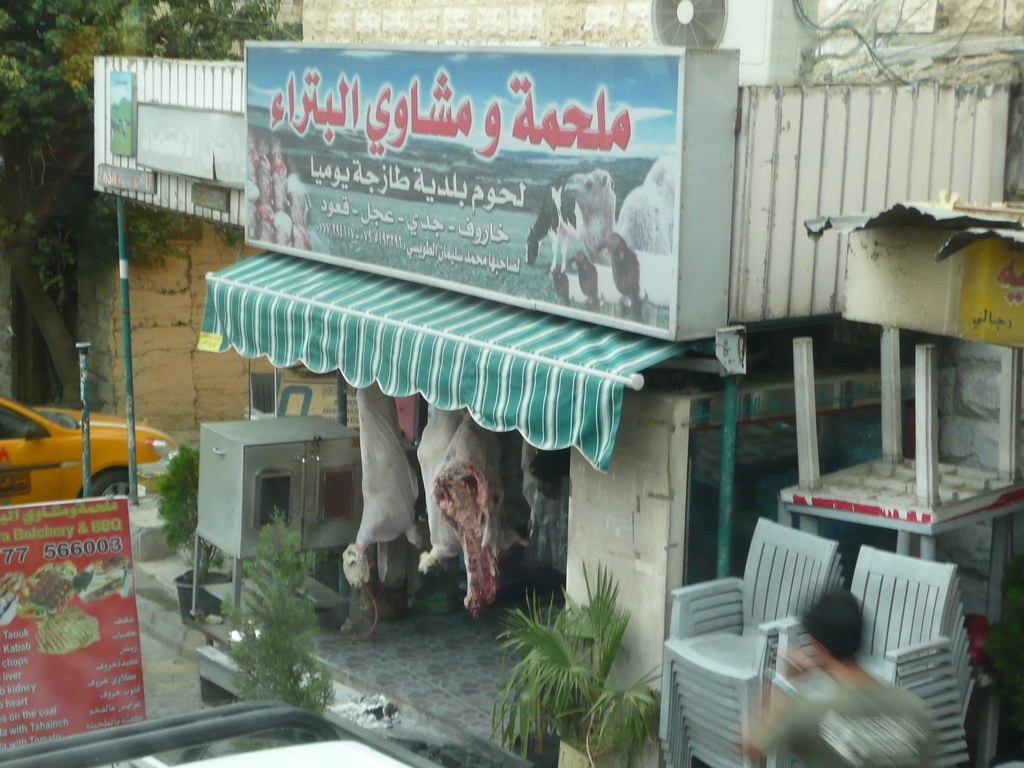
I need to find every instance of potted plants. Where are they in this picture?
[501,555,659,768]
[156,442,232,625]
[491,586,562,768]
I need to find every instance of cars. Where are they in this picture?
[0,396,178,507]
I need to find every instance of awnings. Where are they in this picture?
[200,252,709,474]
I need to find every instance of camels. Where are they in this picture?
[563,151,671,266]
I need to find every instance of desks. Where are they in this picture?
[779,325,1023,522]
[777,496,1024,628]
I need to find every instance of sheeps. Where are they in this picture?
[246,136,312,252]
[341,380,423,589]
[417,407,530,573]
[548,231,670,323]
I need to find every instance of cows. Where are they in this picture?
[524,165,594,275]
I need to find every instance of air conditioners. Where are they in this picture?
[645,0,819,86]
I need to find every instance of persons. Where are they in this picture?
[752,587,942,768]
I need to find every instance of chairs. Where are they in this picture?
[658,514,977,768]
